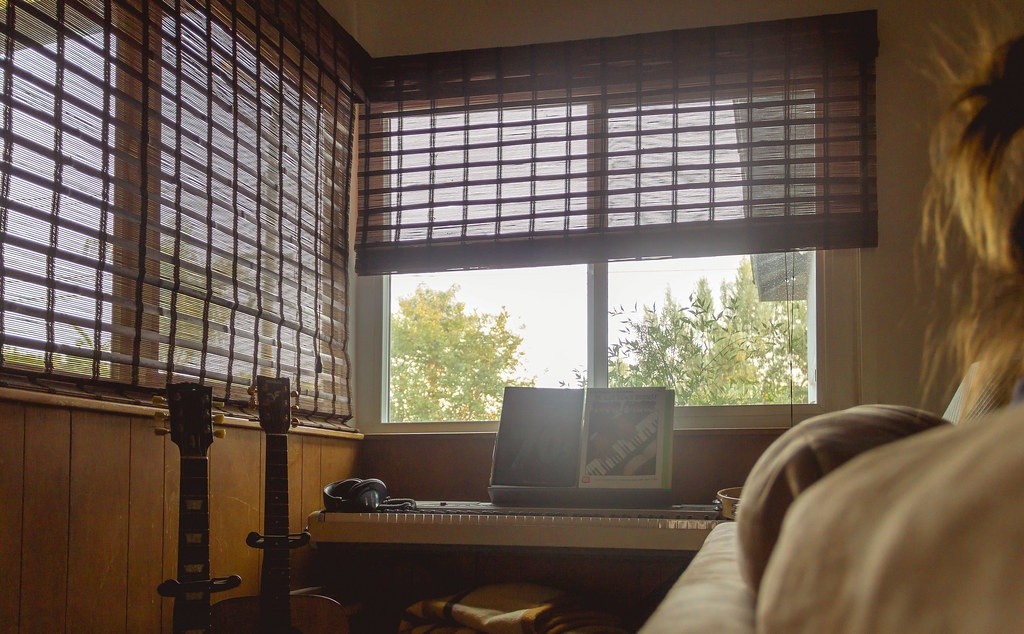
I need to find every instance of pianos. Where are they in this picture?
[305,499,729,551]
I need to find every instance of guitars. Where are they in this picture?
[207,373,354,633]
[149,380,241,634]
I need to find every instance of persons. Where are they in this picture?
[756,22,1024,634]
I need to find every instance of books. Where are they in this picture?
[490,387,677,489]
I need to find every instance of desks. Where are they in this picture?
[311,490,717,551]
